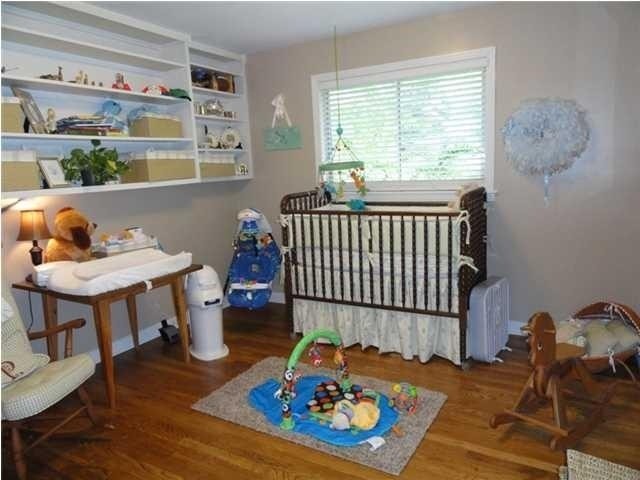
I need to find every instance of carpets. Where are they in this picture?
[189,356,447,476]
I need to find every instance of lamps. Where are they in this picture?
[16,209,53,282]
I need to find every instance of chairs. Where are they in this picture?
[1,263,116,480]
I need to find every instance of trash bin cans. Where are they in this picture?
[184,265,229,361]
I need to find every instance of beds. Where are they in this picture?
[282,186,488,369]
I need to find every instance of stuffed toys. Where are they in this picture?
[44,207,97,262]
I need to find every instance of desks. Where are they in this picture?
[12,263,203,409]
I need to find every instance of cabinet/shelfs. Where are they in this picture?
[188,41,254,183]
[1,1,197,199]
